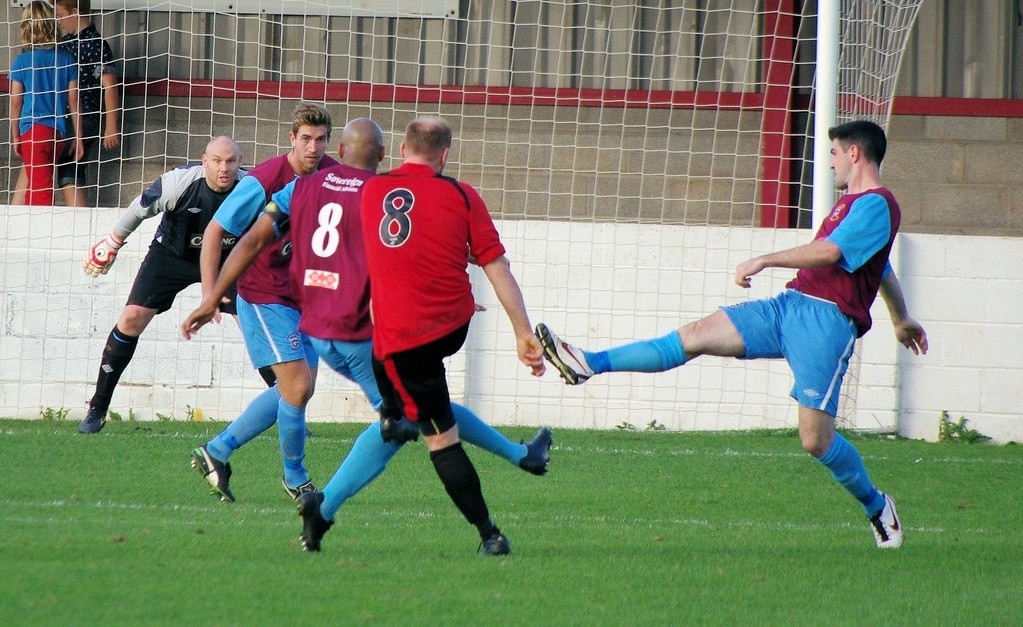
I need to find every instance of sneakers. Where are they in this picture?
[78,406,107,435]
[191,444,235,505]
[535,323,594,385]
[482,532,512,554]
[380,415,420,444]
[867,491,904,549]
[519,425,552,475]
[280,475,317,499]
[296,492,333,553]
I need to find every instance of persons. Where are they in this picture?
[79,107,553,558]
[534,120,928,550]
[6,0,120,208]
